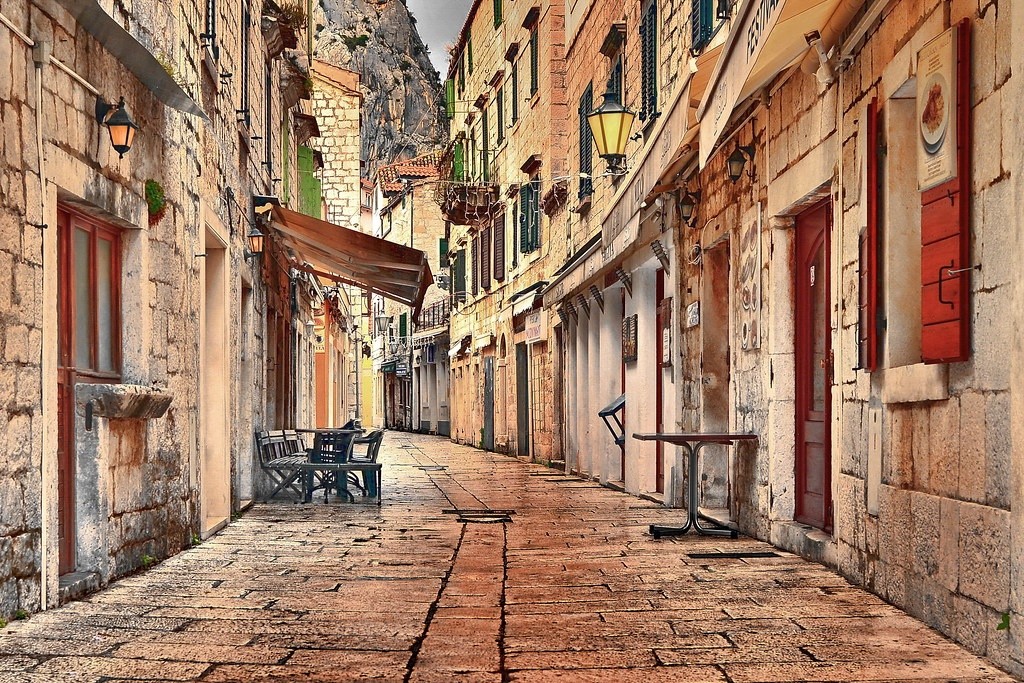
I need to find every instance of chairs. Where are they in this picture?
[255,428,385,506]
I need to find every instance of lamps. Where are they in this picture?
[681,188,701,227]
[244,228,264,263]
[584,89,636,176]
[725,141,755,185]
[94,95,136,158]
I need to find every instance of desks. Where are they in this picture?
[295,429,363,504]
[321,429,367,497]
[631,434,758,538]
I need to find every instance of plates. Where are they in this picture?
[920,73,948,144]
[920,122,946,153]
